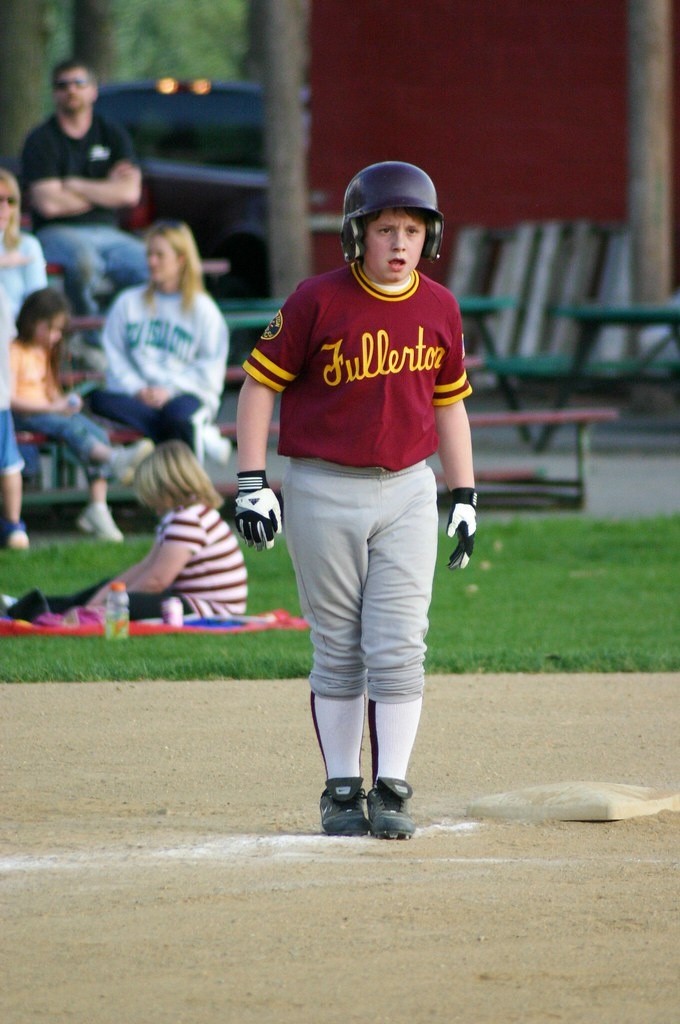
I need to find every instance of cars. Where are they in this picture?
[99,78,269,296]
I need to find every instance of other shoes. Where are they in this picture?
[198,423,233,467]
[5,520,27,548]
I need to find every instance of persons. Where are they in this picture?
[0,61,248,631]
[236,161,478,842]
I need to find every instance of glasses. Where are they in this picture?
[0,194,17,206]
[51,77,94,88]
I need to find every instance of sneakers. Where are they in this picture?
[366,777,416,839]
[109,438,154,487]
[322,778,369,836]
[78,504,125,543]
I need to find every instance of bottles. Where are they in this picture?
[105,582,131,642]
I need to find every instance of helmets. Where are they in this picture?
[341,161,444,263]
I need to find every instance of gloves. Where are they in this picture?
[235,471,281,549]
[446,487,479,568]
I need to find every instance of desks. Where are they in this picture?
[532,299,680,454]
[449,296,532,445]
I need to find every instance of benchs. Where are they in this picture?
[15,211,680,510]
